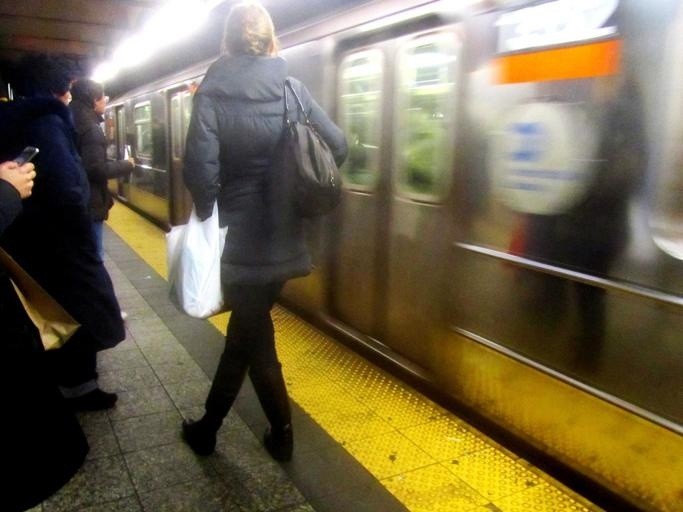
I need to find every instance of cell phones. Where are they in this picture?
[12,146,39,167]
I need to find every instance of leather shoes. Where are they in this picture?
[62,387,118,418]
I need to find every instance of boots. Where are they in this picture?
[249,361,294,462]
[182,353,251,459]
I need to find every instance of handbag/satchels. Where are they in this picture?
[0,246,83,356]
[160,200,232,319]
[278,79,342,216]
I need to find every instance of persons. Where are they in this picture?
[516,69,647,370]
[183,2,349,461]
[0,53,134,512]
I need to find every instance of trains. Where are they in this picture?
[104,3,683,512]
